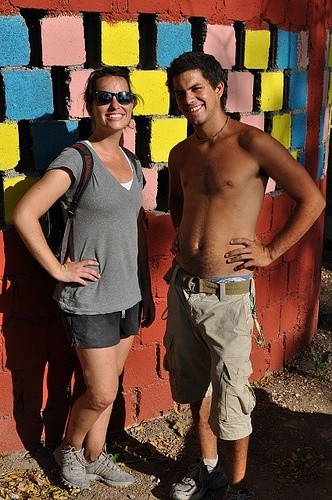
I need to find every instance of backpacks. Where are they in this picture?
[30,144,143,298]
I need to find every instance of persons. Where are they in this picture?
[162,51,327,500]
[11,66,156,490]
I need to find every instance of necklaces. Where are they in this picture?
[194,117,230,142]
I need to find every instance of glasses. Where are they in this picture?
[90,91,136,105]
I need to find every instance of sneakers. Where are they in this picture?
[170,455,227,500]
[223,484,254,500]
[53,443,90,489]
[83,451,135,487]
